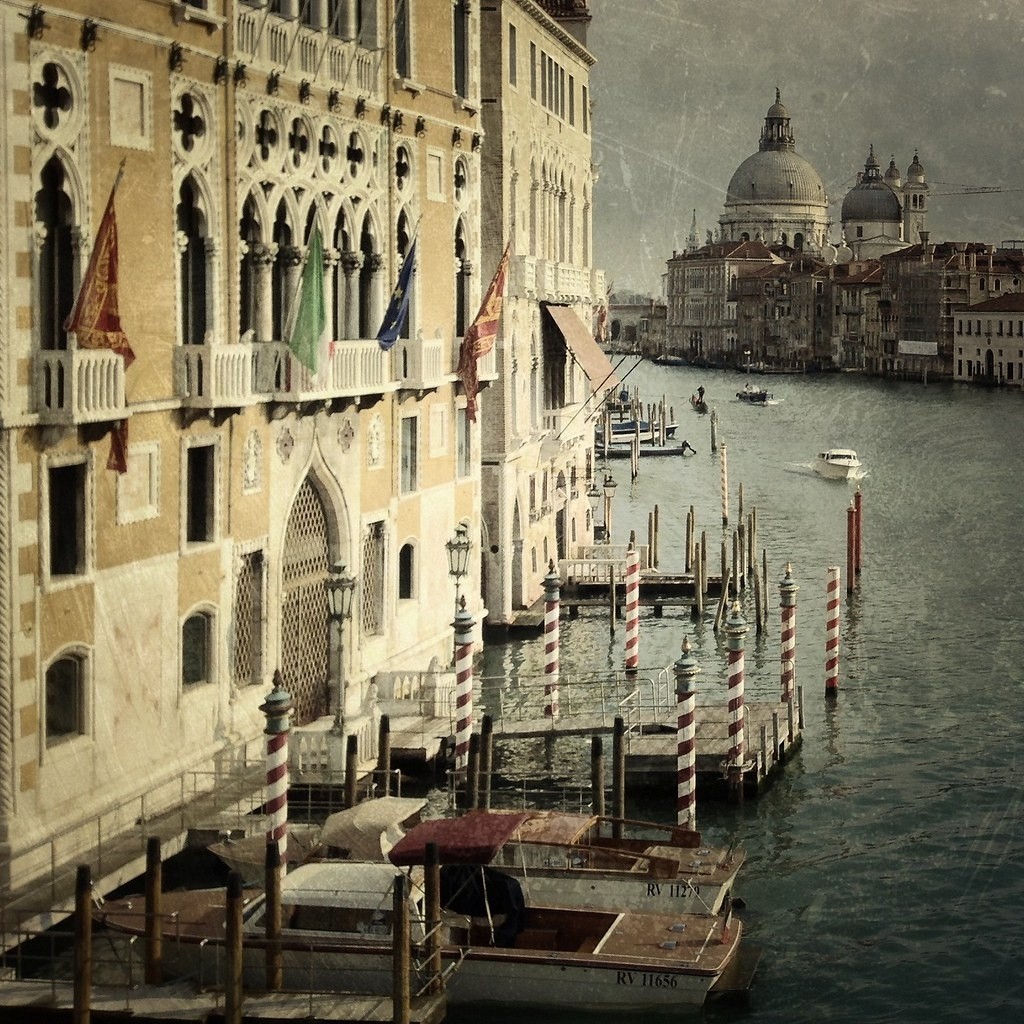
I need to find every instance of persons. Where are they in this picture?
[696,385,704,403]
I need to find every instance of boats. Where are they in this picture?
[812,448,863,479]
[206,797,748,918]
[739,393,773,401]
[691,393,708,414]
[87,862,743,1016]
[595,446,685,455]
[595,418,679,443]
[656,355,688,366]
[606,398,642,408]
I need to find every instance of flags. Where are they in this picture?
[458,250,508,422]
[598,289,611,342]
[71,187,135,474]
[289,224,326,375]
[375,240,416,350]
